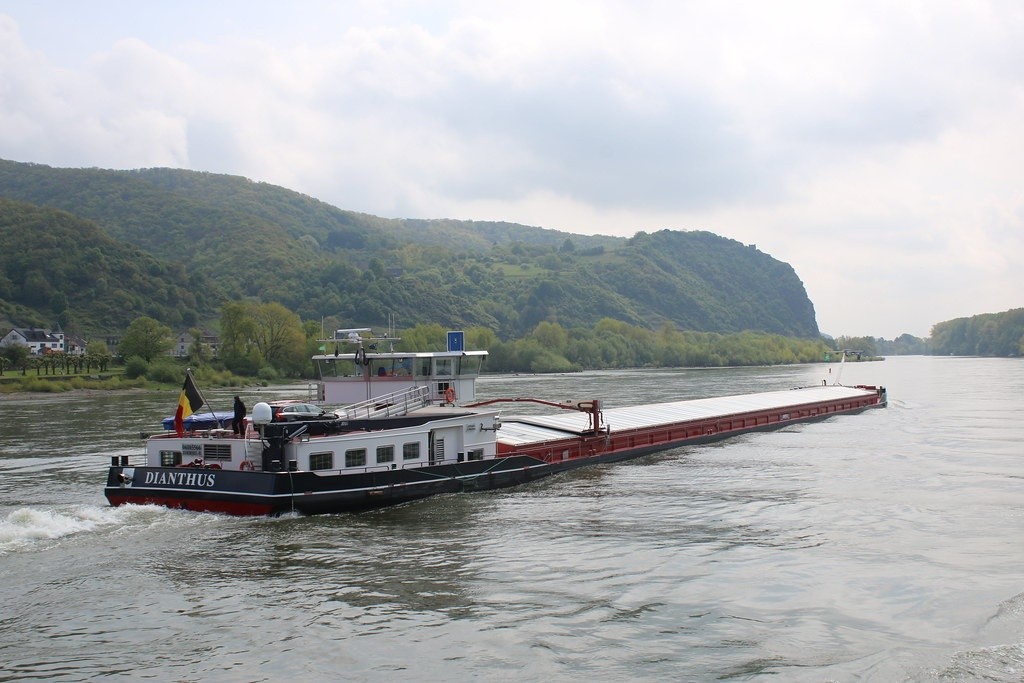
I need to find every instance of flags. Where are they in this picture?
[174,374,204,438]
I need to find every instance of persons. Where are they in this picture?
[232,396,246,437]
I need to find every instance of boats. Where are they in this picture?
[104,328,887,518]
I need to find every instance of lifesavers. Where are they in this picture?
[445,388,455,402]
[240,461,254,470]
